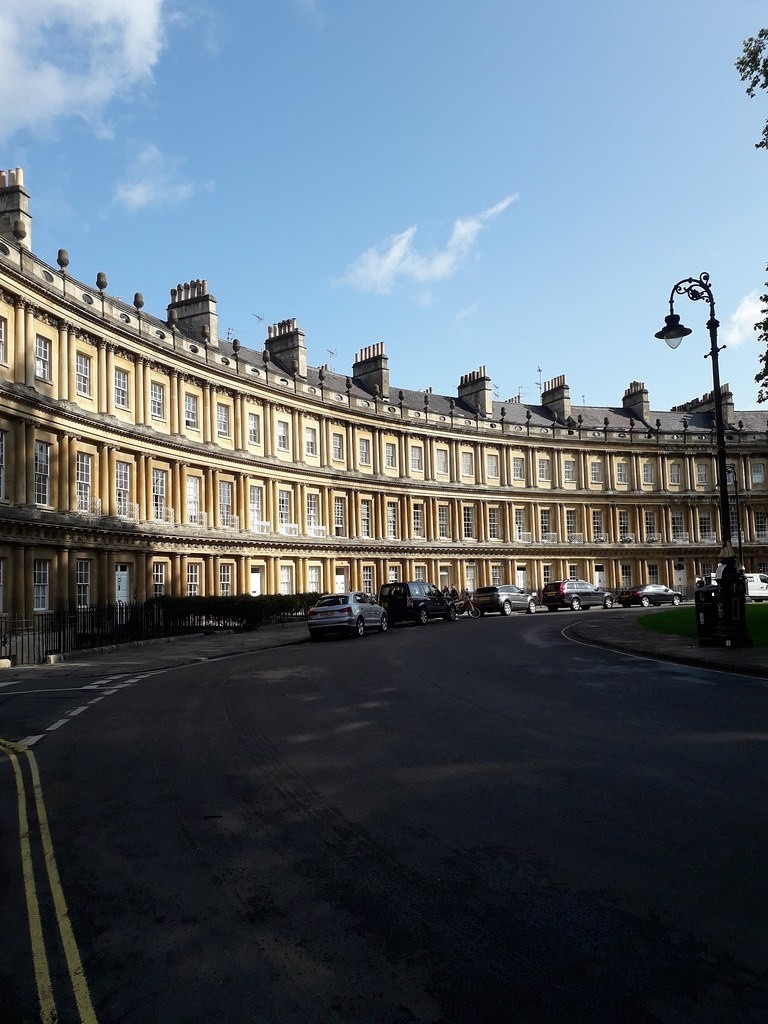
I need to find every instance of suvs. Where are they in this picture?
[379,580,458,627]
[542,578,614,612]
[471,584,537,618]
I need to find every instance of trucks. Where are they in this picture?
[695,573,768,599]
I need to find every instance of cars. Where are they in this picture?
[618,584,683,608]
[307,592,389,638]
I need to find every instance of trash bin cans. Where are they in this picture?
[695,585,718,646]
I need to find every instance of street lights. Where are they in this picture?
[654,274,751,648]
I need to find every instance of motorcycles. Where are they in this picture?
[449,587,482,619]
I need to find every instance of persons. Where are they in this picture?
[443,585,450,598]
[695,577,705,590]
[451,585,459,601]
[466,588,472,600]
